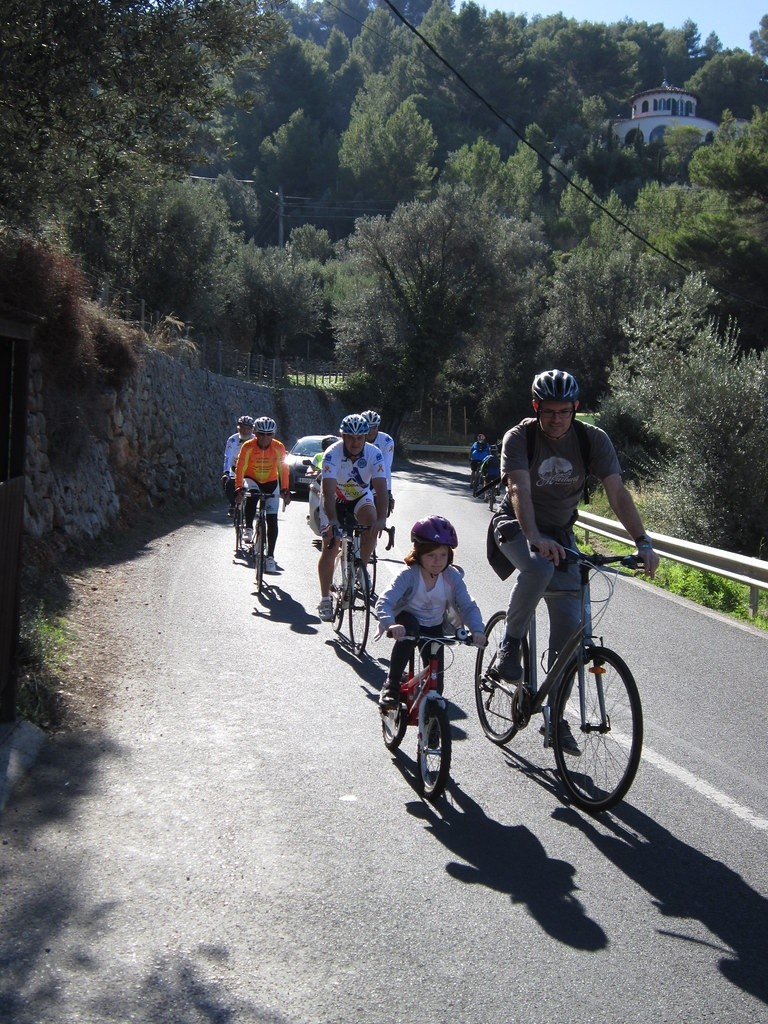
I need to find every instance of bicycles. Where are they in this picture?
[220,460,379,594]
[329,522,395,658]
[470,459,500,511]
[376,629,490,804]
[472,543,654,813]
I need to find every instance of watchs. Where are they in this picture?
[635,534,652,543]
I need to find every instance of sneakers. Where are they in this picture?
[496,640,522,683]
[242,528,253,542]
[265,556,276,573]
[356,567,372,590]
[318,597,333,620]
[539,719,582,756]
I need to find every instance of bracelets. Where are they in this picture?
[638,544,652,549]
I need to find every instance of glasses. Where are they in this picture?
[255,433,274,436]
[538,406,575,419]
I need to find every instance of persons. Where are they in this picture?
[373,515,487,749]
[487,369,658,755]
[234,416,291,572]
[222,416,256,518]
[306,409,395,619]
[470,433,501,501]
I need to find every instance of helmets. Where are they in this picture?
[478,434,485,439]
[321,435,338,450]
[489,445,498,451]
[361,410,381,428]
[411,515,458,549]
[531,369,580,403]
[339,414,369,435]
[238,416,254,426]
[253,417,277,434]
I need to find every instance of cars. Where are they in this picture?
[283,434,341,495]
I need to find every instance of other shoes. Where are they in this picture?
[227,506,236,518]
[483,497,488,503]
[379,681,401,706]
[495,487,500,496]
[426,725,440,750]
[470,483,474,488]
